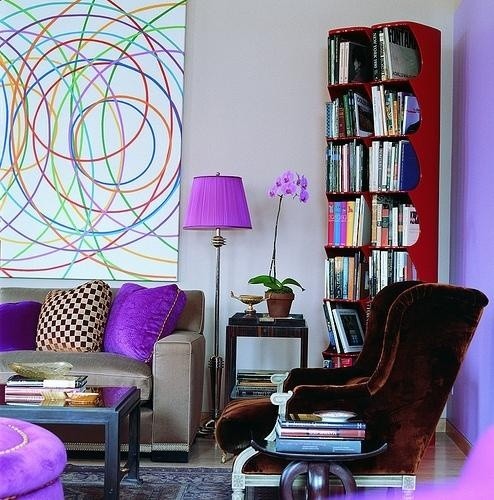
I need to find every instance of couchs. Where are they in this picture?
[0,289,204,463]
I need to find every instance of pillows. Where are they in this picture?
[103,282,190,366]
[33,279,112,356]
[0,301,42,353]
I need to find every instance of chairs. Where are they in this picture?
[214,280,489,500]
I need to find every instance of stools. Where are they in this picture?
[0,415,70,500]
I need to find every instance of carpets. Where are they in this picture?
[61,465,306,500]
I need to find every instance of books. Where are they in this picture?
[322,23,419,358]
[234,367,291,397]
[5,374,101,409]
[273,405,367,454]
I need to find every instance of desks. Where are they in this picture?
[250,430,389,499]
[223,311,309,411]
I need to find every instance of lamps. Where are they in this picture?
[182,172,253,439]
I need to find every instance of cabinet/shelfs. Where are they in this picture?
[321,20,442,368]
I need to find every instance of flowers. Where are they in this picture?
[245,167,310,294]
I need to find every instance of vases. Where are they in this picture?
[264,288,295,317]
